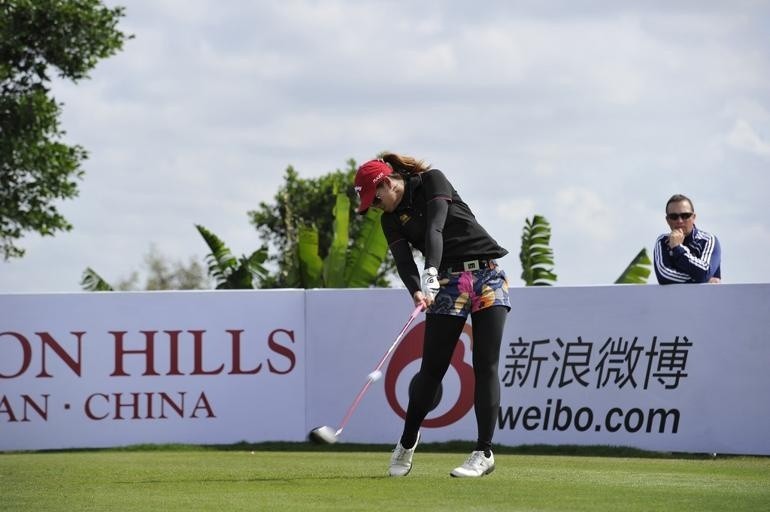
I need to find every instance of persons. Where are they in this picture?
[653,195,722,284]
[354,151,512,479]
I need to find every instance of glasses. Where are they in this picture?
[372,192,382,207]
[667,213,694,221]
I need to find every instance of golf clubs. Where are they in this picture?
[308,304,425,446]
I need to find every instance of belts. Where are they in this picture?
[450,261,488,273]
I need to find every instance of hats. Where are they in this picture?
[354,159,394,214]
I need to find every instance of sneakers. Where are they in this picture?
[450,449,496,477]
[389,431,422,477]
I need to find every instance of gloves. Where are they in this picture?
[421,267,441,302]
